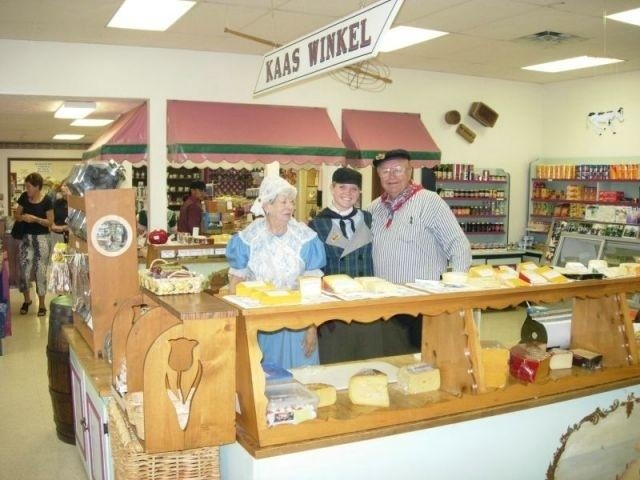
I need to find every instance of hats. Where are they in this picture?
[373,149,411,167]
[190,181,206,190]
[333,168,362,185]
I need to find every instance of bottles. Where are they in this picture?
[438,188,505,198]
[460,220,504,233]
[130,167,206,207]
[433,163,506,183]
[535,163,640,179]
[447,199,506,216]
[207,169,253,198]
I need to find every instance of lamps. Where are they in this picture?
[53,102,96,120]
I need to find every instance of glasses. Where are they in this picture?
[379,166,409,178]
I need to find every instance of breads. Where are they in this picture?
[441,261,568,286]
[300,275,321,298]
[401,363,442,394]
[323,270,393,294]
[346,368,391,407]
[236,280,302,303]
[305,384,337,407]
[482,340,602,390]
[564,260,640,280]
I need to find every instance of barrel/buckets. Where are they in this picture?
[44,296,76,445]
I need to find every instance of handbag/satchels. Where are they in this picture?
[11,220,27,240]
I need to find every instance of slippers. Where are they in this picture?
[38,307,47,316]
[20,300,32,315]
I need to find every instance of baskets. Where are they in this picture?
[137,259,204,297]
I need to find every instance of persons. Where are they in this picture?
[175,179,206,234]
[51,180,69,247]
[366,147,473,359]
[221,172,328,381]
[308,164,385,366]
[11,172,56,317]
